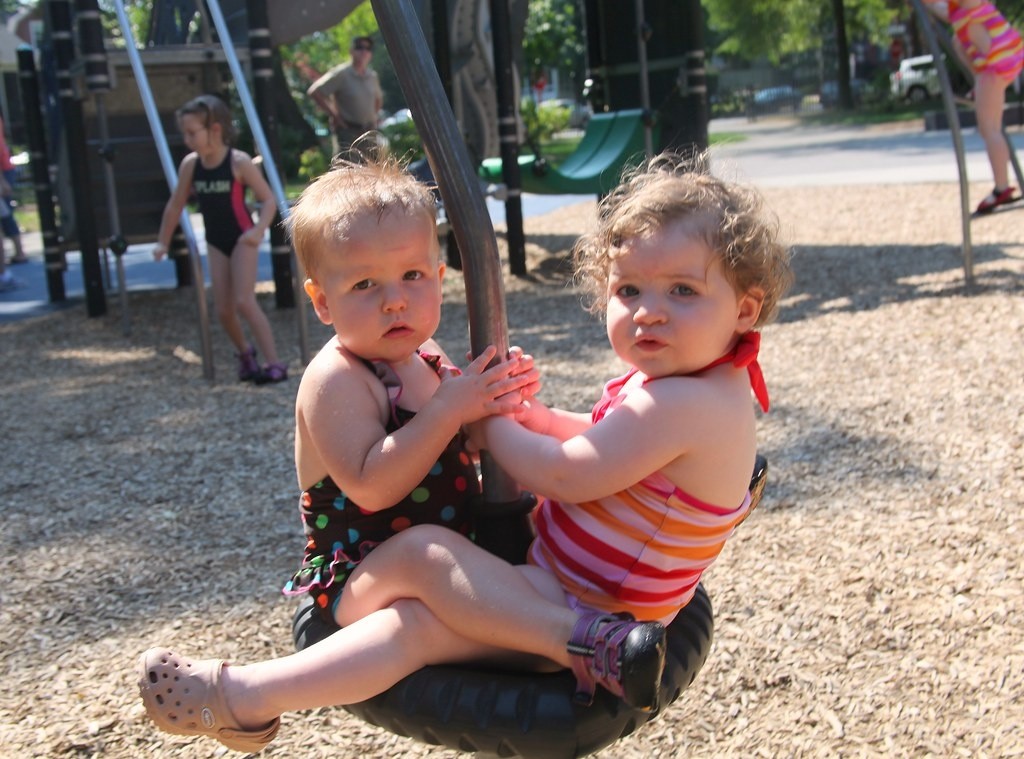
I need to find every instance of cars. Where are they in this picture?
[819,77,868,107]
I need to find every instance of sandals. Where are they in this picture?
[734,453,767,530]
[973,186,1018,215]
[136,647,279,753]
[567,610,668,715]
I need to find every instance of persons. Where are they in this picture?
[917,0,1024,215]
[305,36,383,165]
[138,125,800,752]
[153,94,290,387]
[0,118,32,294]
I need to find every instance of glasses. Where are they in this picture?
[353,44,372,51]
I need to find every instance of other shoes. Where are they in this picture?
[254,362,288,384]
[234,347,258,380]
[6,257,28,264]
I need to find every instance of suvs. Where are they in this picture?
[888,54,946,108]
